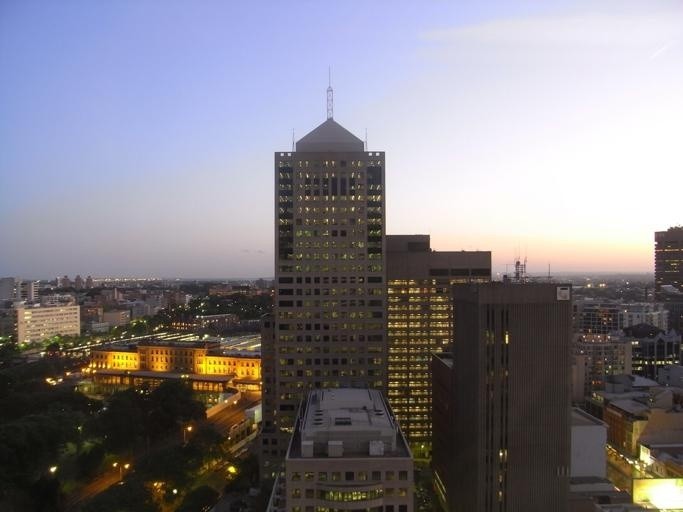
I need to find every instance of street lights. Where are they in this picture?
[182,426,191,444]
[223,399,238,420]
[112,463,129,483]
